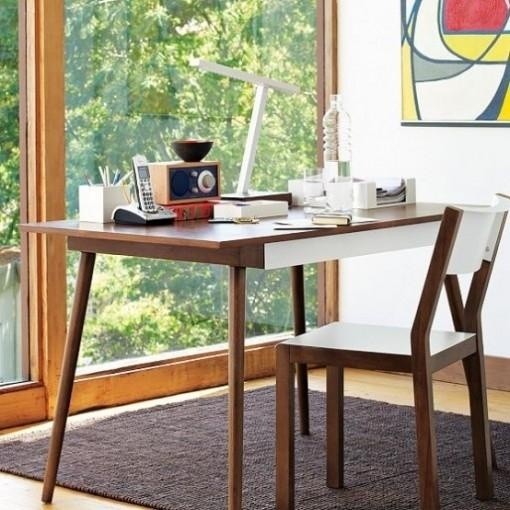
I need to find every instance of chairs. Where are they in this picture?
[274,192,510,507]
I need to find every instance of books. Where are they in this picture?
[213,199,288,218]
[312,213,352,225]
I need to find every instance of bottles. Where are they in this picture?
[322,95,353,214]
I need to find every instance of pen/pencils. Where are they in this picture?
[207,200,240,204]
[87,165,134,186]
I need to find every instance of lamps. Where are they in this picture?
[188,58,301,209]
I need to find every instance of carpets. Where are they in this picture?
[0,384,510,510]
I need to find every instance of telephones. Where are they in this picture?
[111,153,177,226]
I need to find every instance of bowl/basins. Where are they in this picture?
[171,140,212,162]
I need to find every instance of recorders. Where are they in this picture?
[147,162,221,205]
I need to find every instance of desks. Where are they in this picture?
[18,201,490,510]
